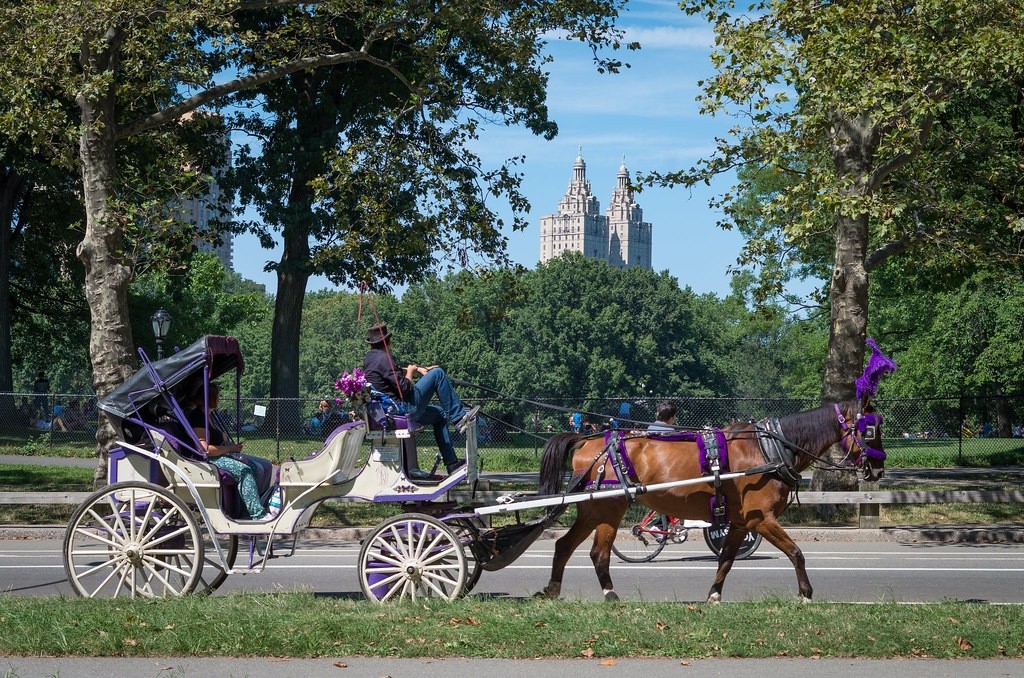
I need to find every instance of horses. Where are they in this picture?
[536,393,886,603]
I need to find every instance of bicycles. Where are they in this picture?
[609,503,763,564]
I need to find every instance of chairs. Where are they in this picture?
[362,400,410,439]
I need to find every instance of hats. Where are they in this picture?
[365,325,392,344]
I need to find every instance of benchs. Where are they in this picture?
[150,429,247,514]
[275,420,366,487]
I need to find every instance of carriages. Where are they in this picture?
[60,334,900,603]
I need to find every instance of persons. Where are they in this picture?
[363,322,481,476]
[567,413,647,433]
[310,394,362,436]
[451,413,512,445]
[1010,421,1024,439]
[646,402,678,545]
[978,421,999,437]
[174,375,274,521]
[17,371,97,433]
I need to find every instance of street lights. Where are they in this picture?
[150,306,174,421]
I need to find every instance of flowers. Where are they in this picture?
[334,367,371,408]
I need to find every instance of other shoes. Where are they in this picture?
[649,526,670,545]
[259,512,275,522]
[455,405,481,433]
[447,459,467,476]
